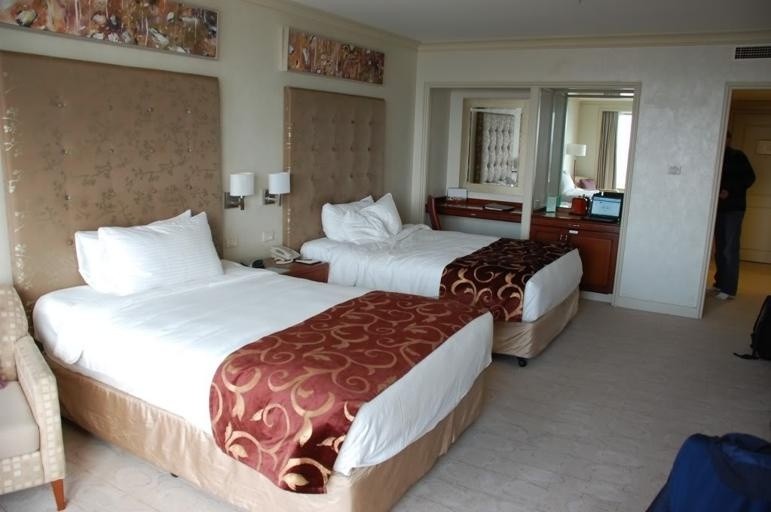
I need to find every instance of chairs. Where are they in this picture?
[427,194,444,232]
[1,284,66,512]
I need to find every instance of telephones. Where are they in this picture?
[270,245,300,261]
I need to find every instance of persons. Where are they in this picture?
[713,128,755,301]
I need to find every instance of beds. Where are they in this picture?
[30,259,493,512]
[301,223,583,368]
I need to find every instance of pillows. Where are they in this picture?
[72,209,224,298]
[321,192,403,246]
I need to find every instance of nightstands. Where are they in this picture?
[260,256,329,283]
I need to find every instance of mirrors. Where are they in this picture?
[445,88,634,208]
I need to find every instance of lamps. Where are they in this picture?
[223,171,290,211]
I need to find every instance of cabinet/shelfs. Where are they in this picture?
[528,206,620,305]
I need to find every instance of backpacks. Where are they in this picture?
[734,295,771,359]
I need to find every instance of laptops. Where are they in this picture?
[584,193,623,223]
